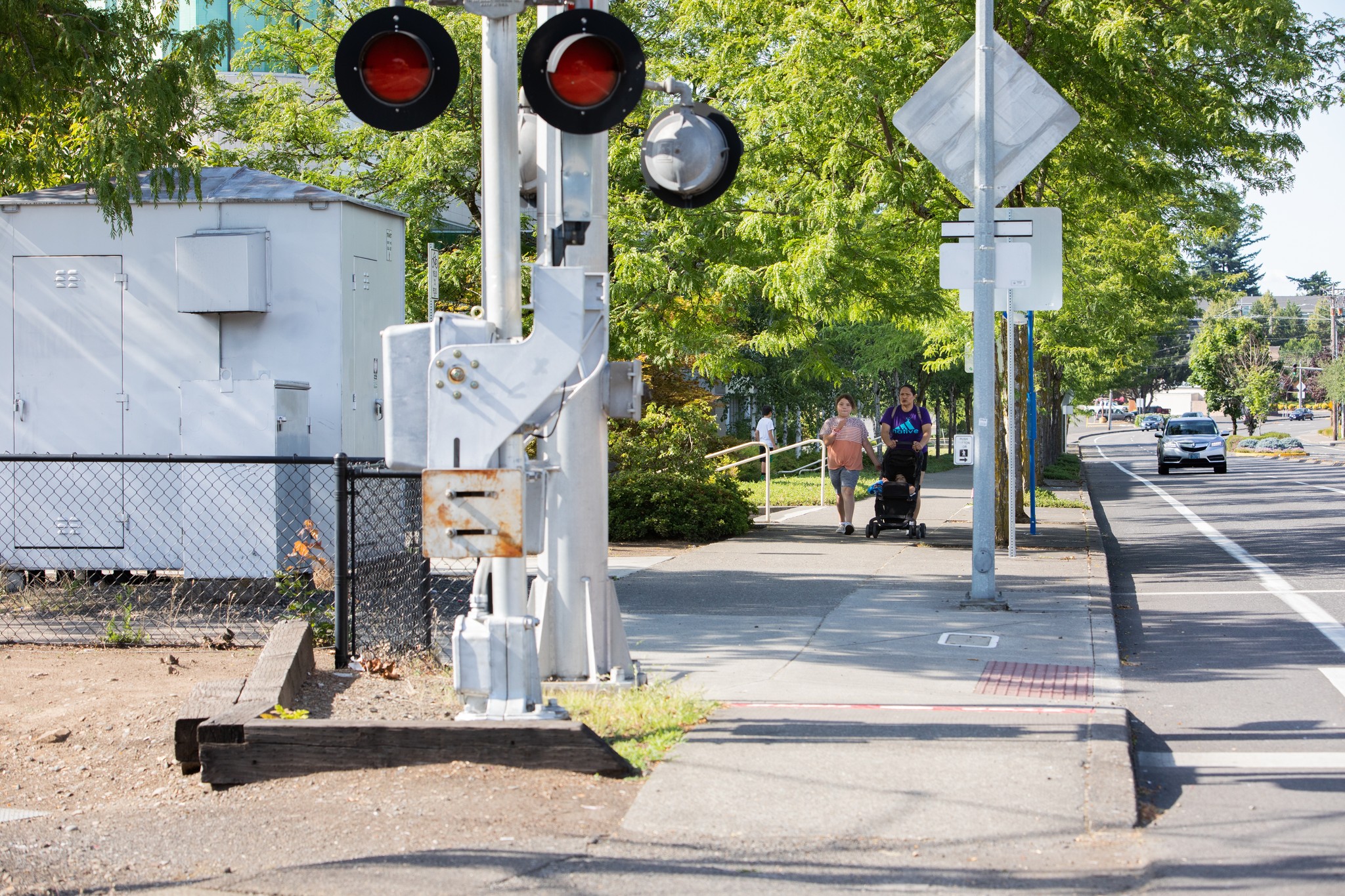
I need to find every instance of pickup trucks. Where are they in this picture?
[1084,400,1128,415]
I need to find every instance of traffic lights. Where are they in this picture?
[1293,364,1297,375]
[521,7,647,135]
[336,4,460,131]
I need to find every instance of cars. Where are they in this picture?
[1131,406,1170,416]
[1243,414,1266,424]
[1289,408,1314,421]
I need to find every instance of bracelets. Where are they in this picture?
[836,428,842,433]
[832,429,841,436]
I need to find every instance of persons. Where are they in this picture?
[754,405,778,482]
[881,473,916,496]
[879,384,933,537]
[819,393,882,535]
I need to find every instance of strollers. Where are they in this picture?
[865,441,927,539]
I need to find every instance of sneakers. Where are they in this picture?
[906,522,913,536]
[843,523,855,535]
[835,524,845,533]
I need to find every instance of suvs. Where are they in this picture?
[1182,412,1204,418]
[1096,408,1135,422]
[1141,415,1165,432]
[1155,416,1229,475]
